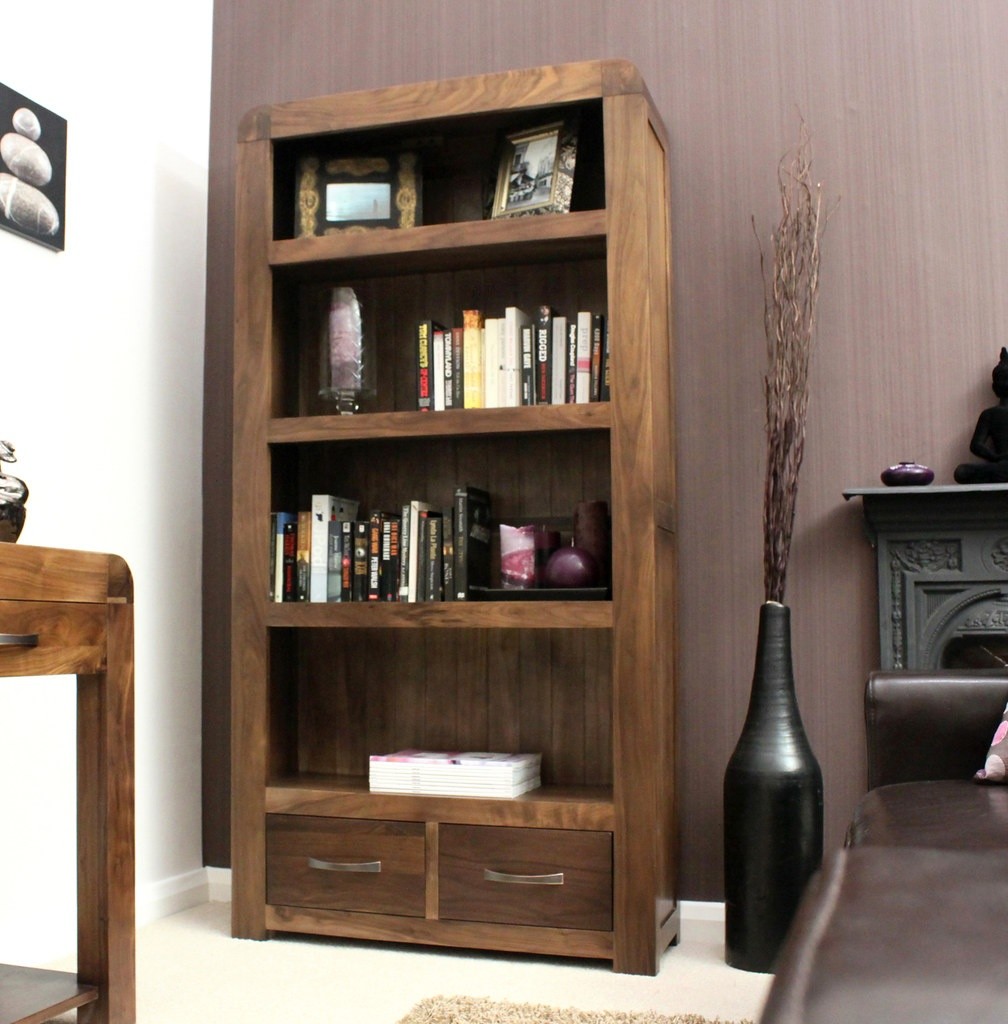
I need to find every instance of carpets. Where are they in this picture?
[394,989,761,1023]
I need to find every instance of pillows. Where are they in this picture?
[972,702,1008,782]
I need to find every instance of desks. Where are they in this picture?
[0,540,134,1023]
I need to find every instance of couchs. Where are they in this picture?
[768,664,1008,1021]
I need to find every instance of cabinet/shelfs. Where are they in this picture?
[229,55,686,977]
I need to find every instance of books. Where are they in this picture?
[415,305,611,412]
[269,486,489,603]
[369,747,542,798]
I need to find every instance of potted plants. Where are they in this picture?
[723,104,827,975]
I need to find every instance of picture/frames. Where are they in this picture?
[295,155,423,239]
[490,119,578,218]
